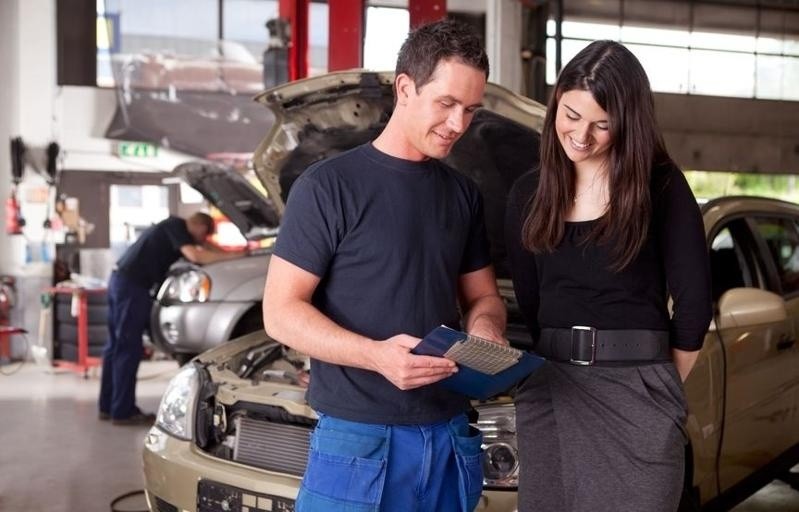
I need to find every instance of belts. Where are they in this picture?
[537,324,670,367]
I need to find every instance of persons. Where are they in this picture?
[506,38,712,512]
[98,213,260,425]
[49,199,87,231]
[263,22,510,512]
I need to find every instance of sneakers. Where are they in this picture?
[99,410,156,426]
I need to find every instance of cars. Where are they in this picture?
[141,67,799,512]
[109,27,277,154]
[145,159,283,369]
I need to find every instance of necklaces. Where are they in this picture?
[574,182,597,204]
